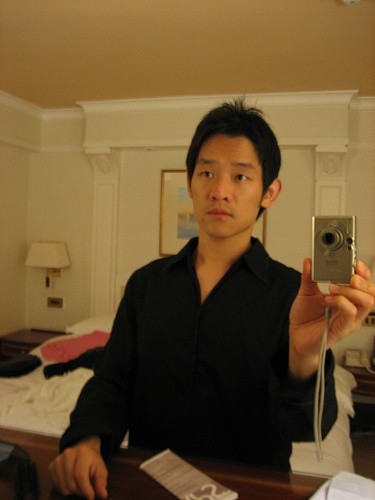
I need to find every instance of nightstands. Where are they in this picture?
[340,353,374,398]
[0,329,72,362]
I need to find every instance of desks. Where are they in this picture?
[0,428,333,500]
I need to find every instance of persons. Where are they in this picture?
[47,100,375,500]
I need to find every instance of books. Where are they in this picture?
[50,449,240,500]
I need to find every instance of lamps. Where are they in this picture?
[25,242,70,288]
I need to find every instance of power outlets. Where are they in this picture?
[360,311,374,326]
[46,297,64,309]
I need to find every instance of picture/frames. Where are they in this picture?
[159,169,267,260]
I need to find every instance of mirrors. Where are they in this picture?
[0,0,375,500]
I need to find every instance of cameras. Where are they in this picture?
[312,215,357,286]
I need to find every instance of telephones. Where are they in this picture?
[343,348,375,375]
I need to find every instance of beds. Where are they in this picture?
[0,313,355,478]
